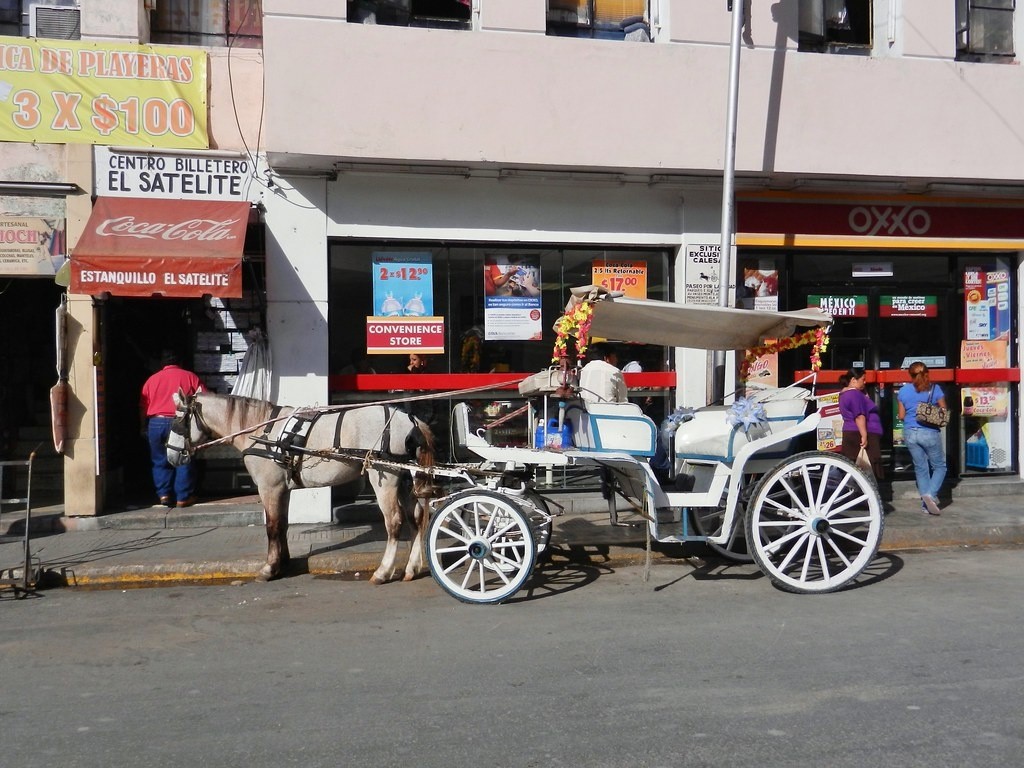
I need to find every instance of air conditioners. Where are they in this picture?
[28,3,80,40]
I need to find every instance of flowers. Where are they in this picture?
[740,327,829,384]
[726,395,767,433]
[551,302,596,364]
[663,406,695,437]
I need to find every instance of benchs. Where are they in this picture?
[565,398,658,457]
[674,388,811,463]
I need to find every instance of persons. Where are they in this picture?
[432,325,496,468]
[897,361,948,515]
[137,349,210,506]
[579,343,628,403]
[484,254,519,296]
[838,367,884,503]
[405,353,431,373]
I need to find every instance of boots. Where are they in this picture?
[652,466,676,491]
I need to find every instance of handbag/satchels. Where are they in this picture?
[845,446,878,488]
[916,383,951,428]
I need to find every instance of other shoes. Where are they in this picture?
[160,496,177,506]
[922,494,940,515]
[177,497,198,507]
[921,505,929,513]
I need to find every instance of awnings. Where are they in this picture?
[64,194,252,298]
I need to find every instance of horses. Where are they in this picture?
[166,385,434,585]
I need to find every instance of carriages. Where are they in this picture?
[164,286,886,605]
